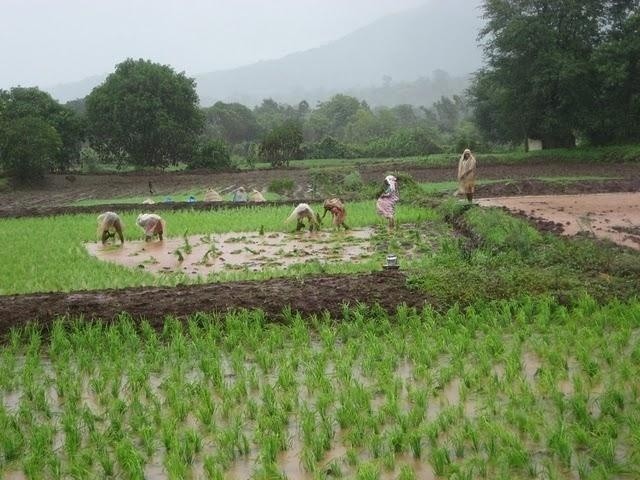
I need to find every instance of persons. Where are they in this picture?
[233,186,247,201]
[135,213,166,242]
[457,148,477,203]
[286,202,317,232]
[96,210,124,245]
[377,175,399,232]
[321,197,349,230]
